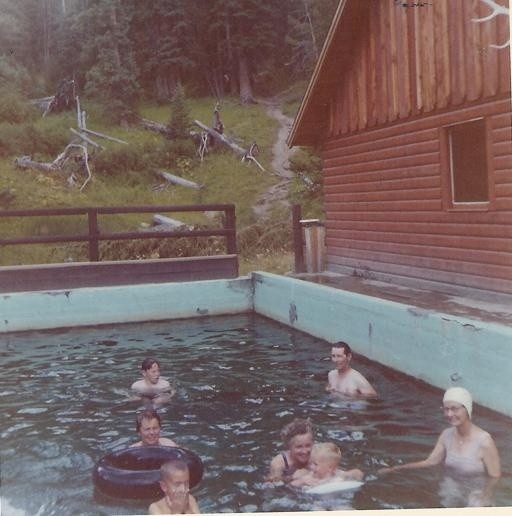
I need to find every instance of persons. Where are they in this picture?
[129,410,180,448]
[290,442,364,511]
[378,387,501,507]
[268,418,317,482]
[131,358,169,405]
[326,342,377,410]
[148,461,199,514]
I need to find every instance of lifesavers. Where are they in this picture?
[93,445,204,499]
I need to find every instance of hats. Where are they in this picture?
[442,387,473,419]
